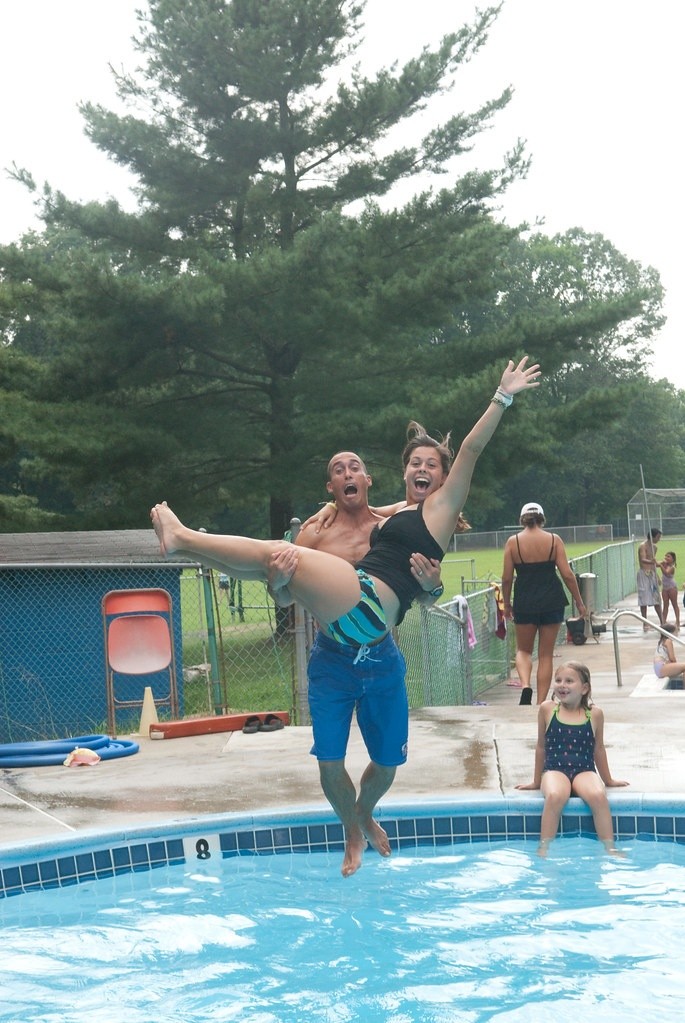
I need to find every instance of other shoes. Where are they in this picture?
[518,687,533,705]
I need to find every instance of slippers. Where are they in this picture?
[259,713,285,732]
[242,715,263,733]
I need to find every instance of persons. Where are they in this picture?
[515,662,630,838]
[149,355,540,647]
[636,528,666,630]
[268,451,444,878]
[654,552,680,628]
[654,623,685,688]
[502,502,587,705]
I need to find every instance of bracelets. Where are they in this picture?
[492,387,514,409]
[327,502,337,510]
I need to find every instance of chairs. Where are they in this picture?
[102,587,179,739]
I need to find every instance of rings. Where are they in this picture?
[419,571,422,575]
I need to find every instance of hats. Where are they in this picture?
[520,502,544,516]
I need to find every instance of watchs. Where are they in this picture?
[430,584,444,596]
